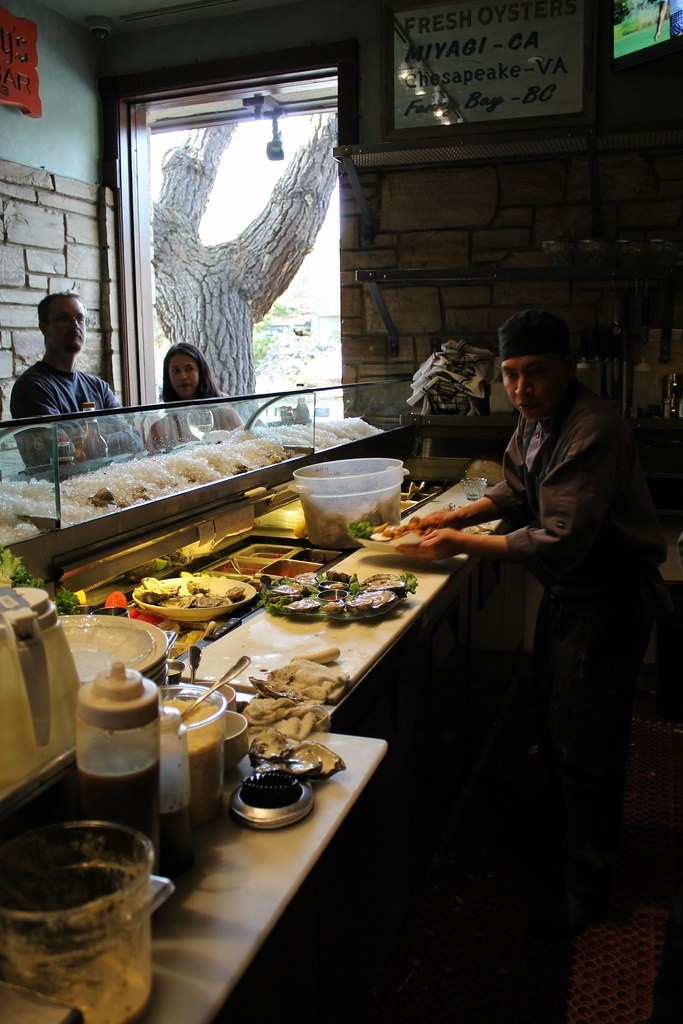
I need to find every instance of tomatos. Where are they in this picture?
[105,592,126,608]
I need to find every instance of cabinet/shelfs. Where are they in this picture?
[333,117,683,363]
[331,527,528,1024]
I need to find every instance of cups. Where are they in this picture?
[195,682,235,712]
[0,822,153,1024]
[91,607,129,619]
[225,711,248,772]
[187,409,214,441]
[461,478,487,500]
[158,685,227,828]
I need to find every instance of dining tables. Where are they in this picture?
[150,732,387,1024]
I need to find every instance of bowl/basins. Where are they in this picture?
[578,240,613,267]
[318,590,348,602]
[650,239,676,264]
[542,241,574,266]
[319,581,349,591]
[167,659,185,684]
[616,240,649,264]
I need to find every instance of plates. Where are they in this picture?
[58,615,169,688]
[279,578,405,619]
[355,538,398,553]
[132,578,256,621]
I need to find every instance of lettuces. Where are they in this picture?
[349,520,373,539]
[259,572,417,615]
[0,543,81,616]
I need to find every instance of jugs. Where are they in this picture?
[1,588,80,789]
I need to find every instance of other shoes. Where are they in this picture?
[525,885,612,938]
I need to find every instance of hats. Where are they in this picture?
[498,309,570,362]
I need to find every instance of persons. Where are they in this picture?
[395,309,668,938]
[145,343,244,453]
[9,292,143,468]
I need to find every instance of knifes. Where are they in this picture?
[177,618,242,662]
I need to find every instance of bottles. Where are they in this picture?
[633,360,651,414]
[76,662,161,874]
[83,402,108,458]
[315,397,329,422]
[577,356,622,401]
[650,374,661,415]
[159,706,192,877]
[295,384,310,425]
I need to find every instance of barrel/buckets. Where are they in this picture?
[289,459,410,548]
